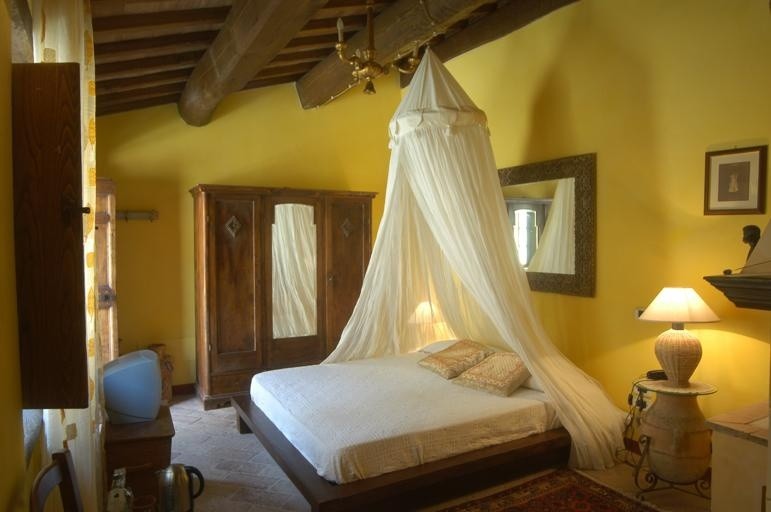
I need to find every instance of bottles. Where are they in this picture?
[108,467,130,512]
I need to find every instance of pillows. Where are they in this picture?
[418,341,457,355]
[417,341,495,379]
[453,351,531,397]
[522,375,544,393]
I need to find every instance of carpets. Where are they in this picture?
[424,467,661,512]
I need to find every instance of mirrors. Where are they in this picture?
[270,202,319,340]
[505,197,553,270]
[497,153,597,297]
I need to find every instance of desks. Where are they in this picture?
[103,405,175,504]
[634,379,719,500]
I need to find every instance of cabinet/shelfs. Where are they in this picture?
[188,184,379,408]
[11,63,87,408]
[705,403,771,512]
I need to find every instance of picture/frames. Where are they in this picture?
[704,144,768,215]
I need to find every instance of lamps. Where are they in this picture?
[638,286,720,386]
[335,1,421,95]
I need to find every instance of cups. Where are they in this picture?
[131,494,158,512]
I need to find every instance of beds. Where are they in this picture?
[231,352,572,512]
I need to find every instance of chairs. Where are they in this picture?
[29,448,83,512]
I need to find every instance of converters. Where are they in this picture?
[647,370,668,380]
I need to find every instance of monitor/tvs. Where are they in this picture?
[103,349,162,426]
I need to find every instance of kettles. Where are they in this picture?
[153,463,205,511]
